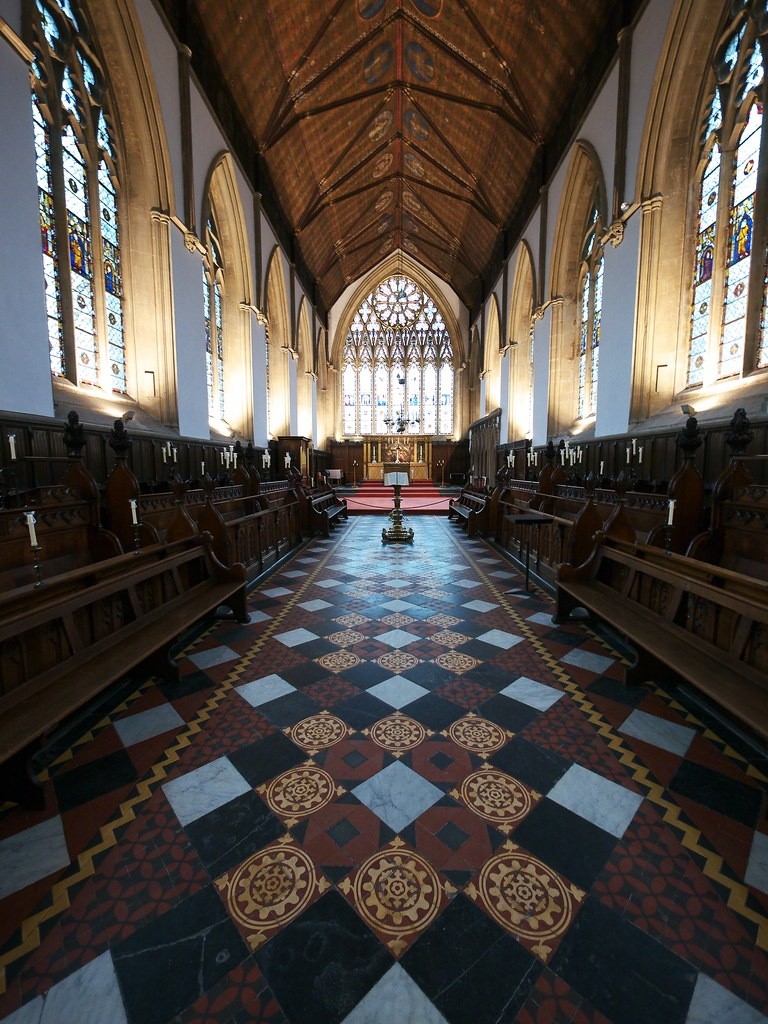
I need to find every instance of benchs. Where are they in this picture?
[448,488,490,538]
[0,530,252,775]
[550,530,768,778]
[305,489,348,539]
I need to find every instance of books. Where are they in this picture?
[383,472,409,487]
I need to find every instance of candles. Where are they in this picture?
[560,442,583,466]
[599,461,604,474]
[507,450,515,468]
[166,441,171,456]
[639,446,644,462]
[8,434,16,459]
[220,445,237,468]
[173,448,177,462]
[632,439,637,455]
[162,447,167,462]
[527,447,538,466]
[262,449,271,468]
[284,453,291,469]
[668,500,676,526]
[626,448,630,463]
[24,511,38,547]
[201,462,205,475]
[131,501,138,525]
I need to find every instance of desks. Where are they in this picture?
[504,514,554,601]
[325,469,343,487]
